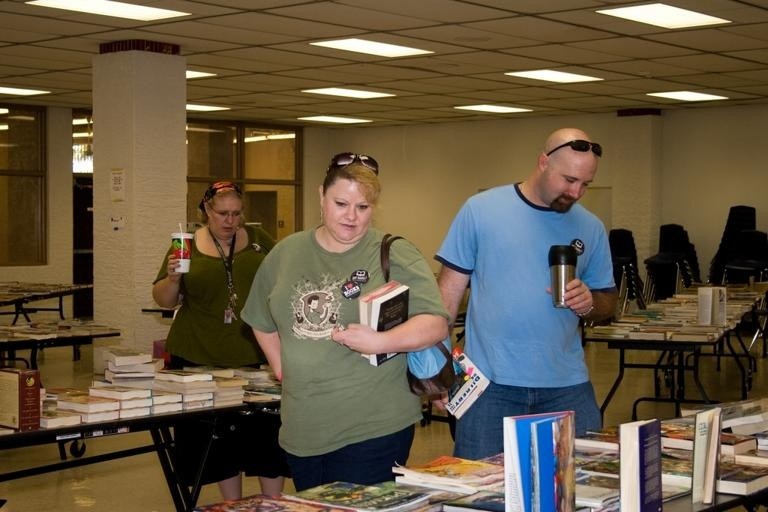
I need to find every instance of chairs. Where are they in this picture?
[609,204,767,313]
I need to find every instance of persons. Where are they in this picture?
[430,126,621,465]
[150,178,293,502]
[236,149,453,495]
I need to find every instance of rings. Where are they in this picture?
[339,340,344,346]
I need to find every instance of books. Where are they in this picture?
[0,316,123,344]
[36,347,282,432]
[591,278,766,341]
[368,284,411,368]
[439,346,490,421]
[357,279,402,361]
[193,395,767,512]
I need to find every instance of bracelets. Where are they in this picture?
[575,303,595,318]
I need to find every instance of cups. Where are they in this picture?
[172,231,192,273]
[549,243,578,308]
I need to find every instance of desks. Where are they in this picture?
[2,280,90,326]
[140,305,181,319]
[2,322,119,374]
[580,283,767,422]
[1,385,279,509]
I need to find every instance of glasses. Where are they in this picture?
[207,202,242,216]
[546,139,603,160]
[327,152,379,176]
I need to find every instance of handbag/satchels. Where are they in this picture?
[402,318,458,400]
